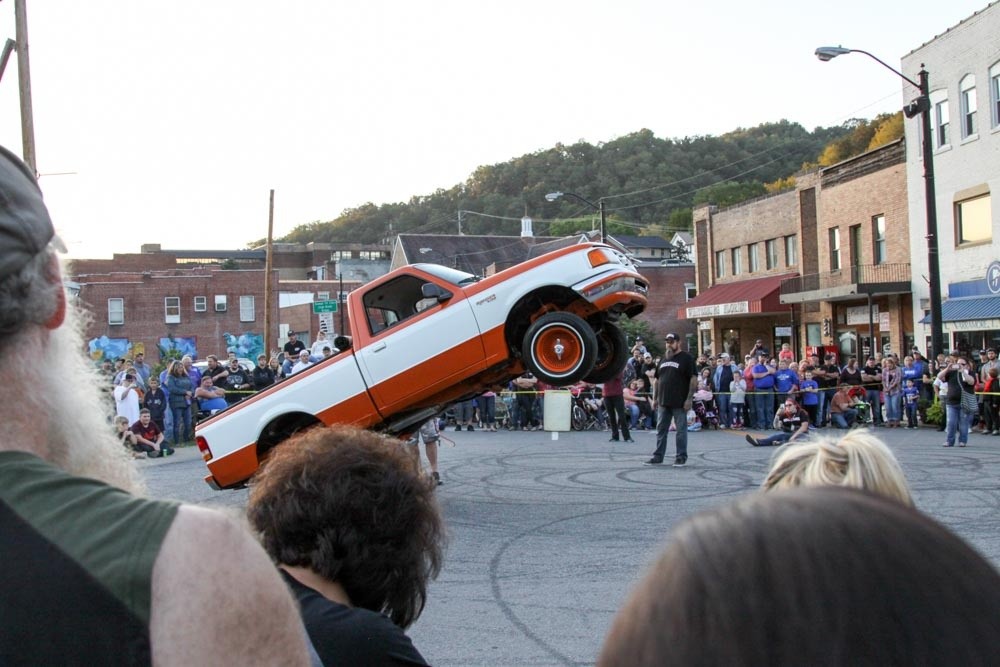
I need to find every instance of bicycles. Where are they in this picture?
[567,387,584,431]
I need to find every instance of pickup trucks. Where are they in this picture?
[194,242,651,491]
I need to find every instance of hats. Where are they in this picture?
[664,333,680,342]
[229,357,237,362]
[719,353,730,358]
[288,330,295,336]
[0,144,54,276]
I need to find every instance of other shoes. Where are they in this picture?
[453,424,544,432]
[967,428,1000,435]
[959,390,979,413]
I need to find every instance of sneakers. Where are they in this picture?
[644,458,663,466]
[772,440,782,445]
[672,458,686,466]
[942,441,955,447]
[958,442,966,447]
[746,434,759,445]
[149,449,175,458]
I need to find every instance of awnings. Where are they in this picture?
[918,296,1000,334]
[678,273,801,320]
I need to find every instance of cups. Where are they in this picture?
[163,449,167,457]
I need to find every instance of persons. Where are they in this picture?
[602,371,635,443]
[1,145,322,667]
[746,396,811,447]
[642,333,698,467]
[94,330,334,460]
[246,421,444,667]
[759,428,916,508]
[439,336,1000,436]
[597,484,1000,667]
[939,356,976,447]
[406,418,443,486]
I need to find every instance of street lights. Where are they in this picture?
[545,190,606,244]
[312,266,344,336]
[815,45,944,361]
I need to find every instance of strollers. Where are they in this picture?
[583,397,609,431]
[848,386,873,424]
[692,389,720,431]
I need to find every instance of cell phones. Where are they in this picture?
[952,364,959,368]
